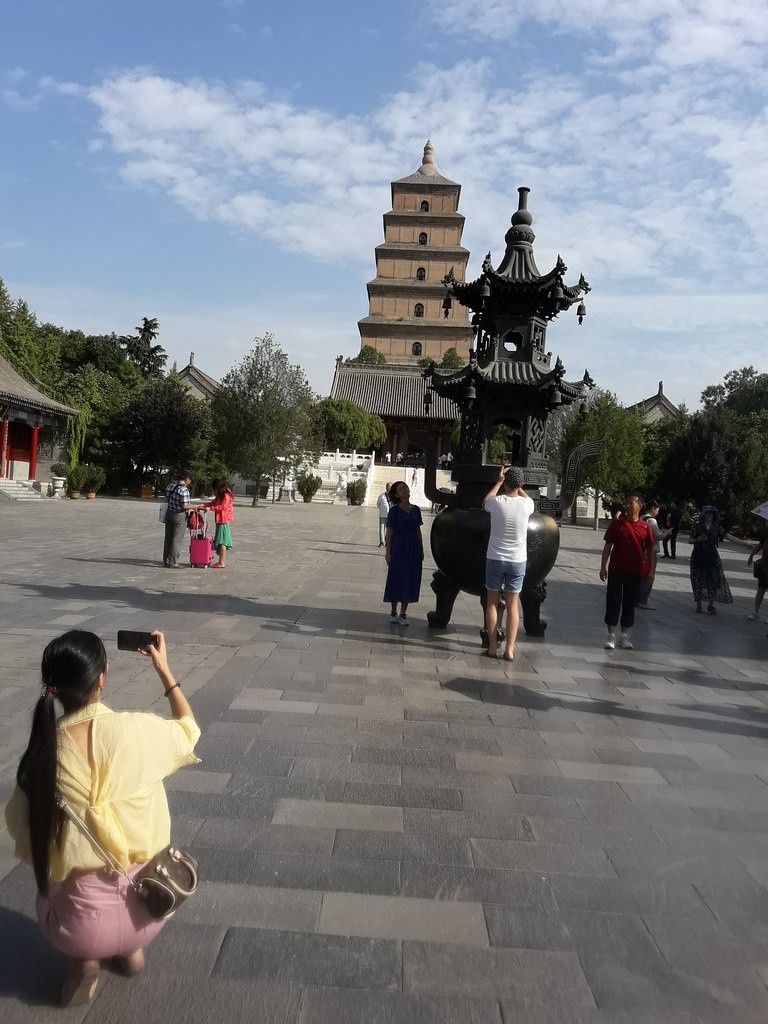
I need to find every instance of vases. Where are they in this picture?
[50,476,66,489]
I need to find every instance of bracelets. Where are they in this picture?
[164,683,180,697]
[744,542,747,546]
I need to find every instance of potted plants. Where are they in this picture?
[49,463,106,499]
[296,473,323,503]
[347,478,368,506]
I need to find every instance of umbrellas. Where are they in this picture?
[750,500,768,520]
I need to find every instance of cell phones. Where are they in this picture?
[117,630,158,653]
[504,465,512,469]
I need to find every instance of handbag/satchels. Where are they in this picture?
[129,844,199,918]
[187,510,204,530]
[159,502,169,523]
[641,560,651,579]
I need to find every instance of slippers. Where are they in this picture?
[503,651,515,660]
[481,648,497,657]
[640,605,657,610]
[210,563,224,568]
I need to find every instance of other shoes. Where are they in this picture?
[398,614,409,626]
[748,612,759,620]
[695,607,702,613]
[378,542,384,548]
[62,958,100,1008]
[390,612,397,623]
[604,636,616,648]
[165,562,186,569]
[660,553,669,559]
[114,949,145,975]
[707,605,716,613]
[617,636,633,649]
[667,554,677,559]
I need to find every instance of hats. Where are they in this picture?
[699,505,719,522]
[181,470,194,485]
[505,467,524,488]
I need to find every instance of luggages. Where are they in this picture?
[189,507,213,569]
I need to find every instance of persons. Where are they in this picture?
[200,478,234,568]
[385,451,454,470]
[600,491,656,649]
[163,470,199,568]
[481,466,534,661]
[688,504,754,615]
[612,502,627,519]
[639,500,672,610]
[661,498,682,559]
[377,482,394,547]
[382,482,424,626]
[747,520,768,623]
[4,630,201,1007]
